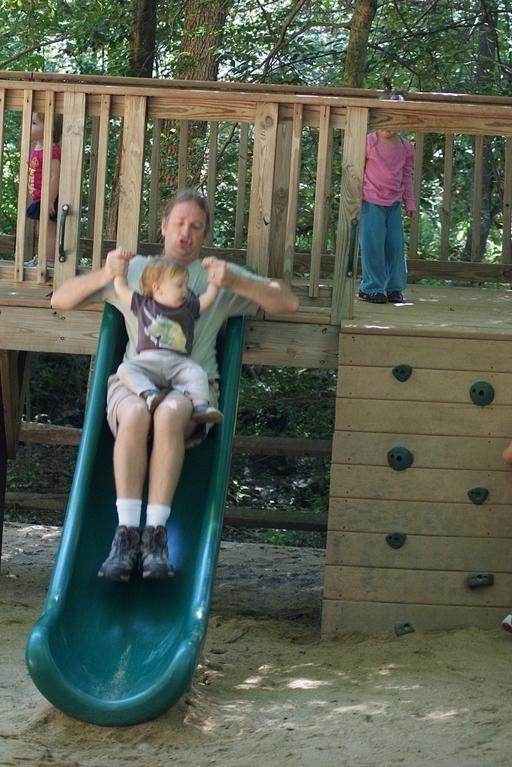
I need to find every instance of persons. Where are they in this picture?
[24,111,62,268]
[502,442,512,631]
[50,193,301,582]
[115,245,225,423]
[358,89,418,301]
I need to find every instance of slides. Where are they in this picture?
[26,263,255,727]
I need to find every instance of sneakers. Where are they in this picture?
[24,255,55,269]
[144,390,166,411]
[98,525,140,590]
[191,406,224,423]
[357,289,386,303]
[387,292,404,302]
[140,527,173,578]
